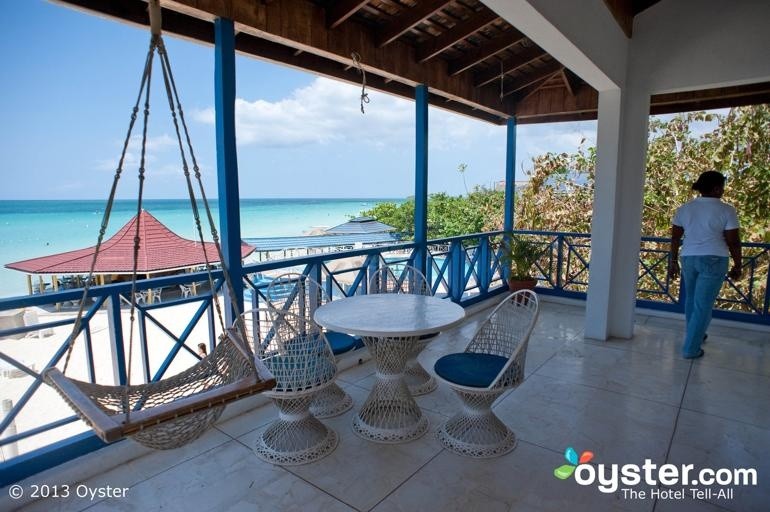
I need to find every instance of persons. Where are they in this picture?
[197,343,208,359]
[667,171,743,359]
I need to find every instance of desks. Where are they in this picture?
[315,294,466,444]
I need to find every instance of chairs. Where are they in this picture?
[179,284,191,297]
[435,290,541,456]
[262,275,356,419]
[232,308,340,465]
[154,287,162,303]
[369,266,437,396]
[133,293,144,305]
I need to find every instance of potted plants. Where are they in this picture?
[499,237,545,306]
